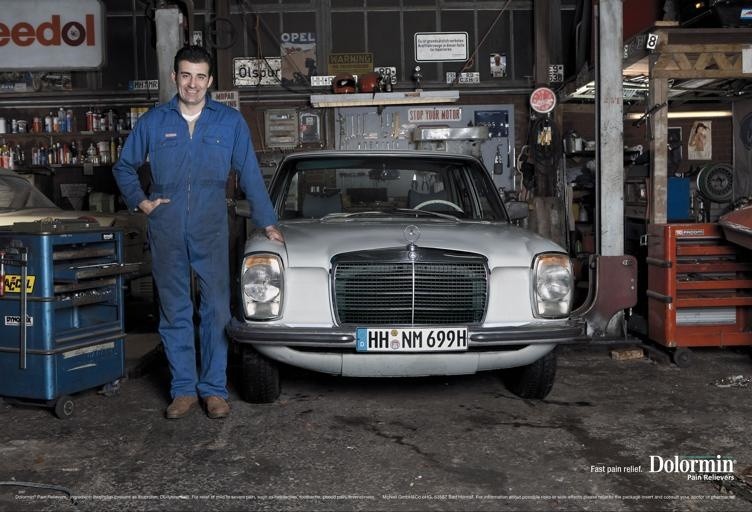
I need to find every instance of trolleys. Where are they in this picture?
[644,221,752,368]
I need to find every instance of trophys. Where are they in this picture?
[413,65,423,92]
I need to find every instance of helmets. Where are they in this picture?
[330,73,355,94]
[357,72,383,93]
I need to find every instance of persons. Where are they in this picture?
[689,122,710,151]
[112,46,284,419]
[491,54,506,77]
[305,57,316,80]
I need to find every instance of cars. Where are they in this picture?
[218,141,590,405]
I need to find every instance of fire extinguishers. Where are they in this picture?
[530,112,558,197]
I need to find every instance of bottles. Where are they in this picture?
[0,108,132,134]
[1,136,125,169]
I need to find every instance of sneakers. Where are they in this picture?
[203,394,229,419]
[165,395,199,419]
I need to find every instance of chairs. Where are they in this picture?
[299,190,348,220]
[407,188,450,211]
